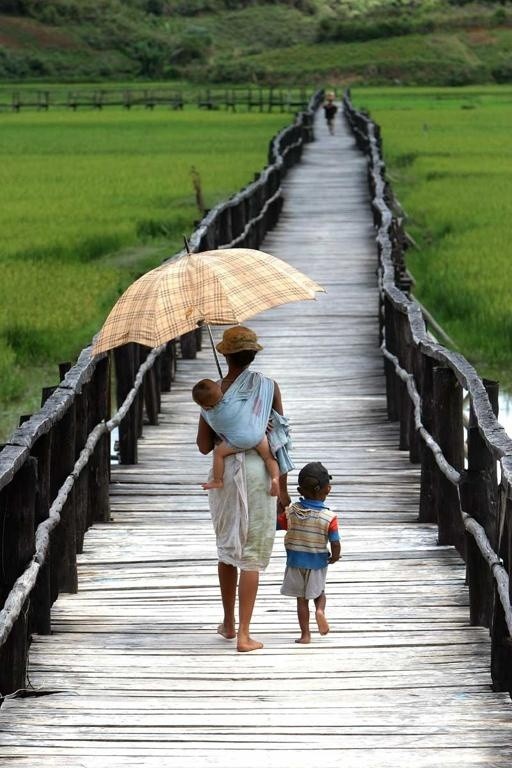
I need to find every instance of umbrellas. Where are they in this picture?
[88,232,324,379]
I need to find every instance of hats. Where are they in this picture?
[214,325,263,355]
[298,460,333,493]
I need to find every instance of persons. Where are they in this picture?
[278,464,342,642]
[183,328,290,653]
[192,378,283,496]
[321,102,337,134]
